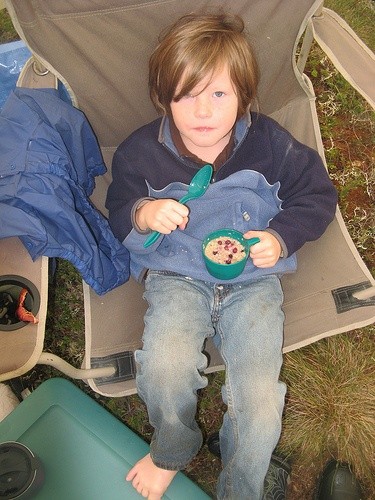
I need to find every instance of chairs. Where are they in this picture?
[0,0,375,397]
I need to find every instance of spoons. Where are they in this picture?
[142,164,213,248]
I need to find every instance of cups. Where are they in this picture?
[0,441,44,500]
[200,229,261,281]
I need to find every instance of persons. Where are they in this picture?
[103,12,337,500]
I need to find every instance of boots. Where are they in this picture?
[319,461,362,500]
[207,433,292,500]
